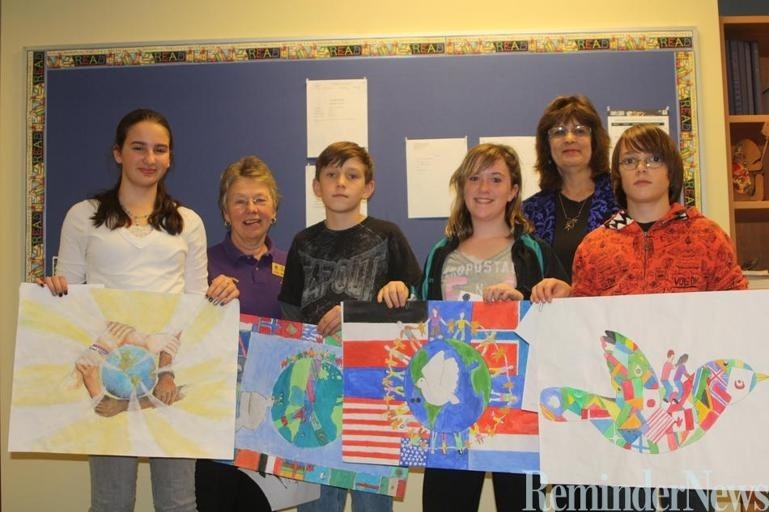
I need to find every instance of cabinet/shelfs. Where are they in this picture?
[720,15,769,275]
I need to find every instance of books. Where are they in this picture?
[723,37,764,116]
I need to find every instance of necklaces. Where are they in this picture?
[114,199,154,238]
[557,191,588,234]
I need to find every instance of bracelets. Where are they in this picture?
[89,344,109,355]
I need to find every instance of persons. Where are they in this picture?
[195,155,293,511]
[529,125,749,510]
[92,383,191,417]
[519,95,620,289]
[34,108,241,512]
[96,319,182,361]
[376,144,547,511]
[151,328,184,406]
[279,141,425,511]
[67,319,136,389]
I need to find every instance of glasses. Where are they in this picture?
[618,153,663,170]
[547,126,591,138]
